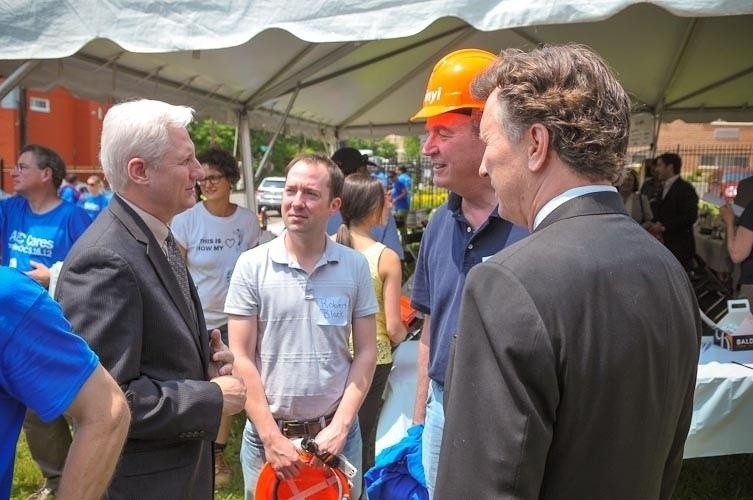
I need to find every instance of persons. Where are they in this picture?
[1,97,413,500]
[717,176,752,307]
[408,44,703,500]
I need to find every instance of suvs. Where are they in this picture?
[255,178,288,217]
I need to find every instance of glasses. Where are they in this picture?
[13,163,42,172]
[196,174,225,186]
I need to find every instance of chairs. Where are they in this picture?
[689,252,736,335]
[403,232,426,275]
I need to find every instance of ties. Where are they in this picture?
[163,227,193,318]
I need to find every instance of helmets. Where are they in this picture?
[254,449,351,500]
[410,48,498,123]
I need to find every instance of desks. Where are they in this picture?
[682,335,753,459]
[693,215,735,281]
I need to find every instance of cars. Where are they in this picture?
[706,163,753,203]
[356,147,431,190]
